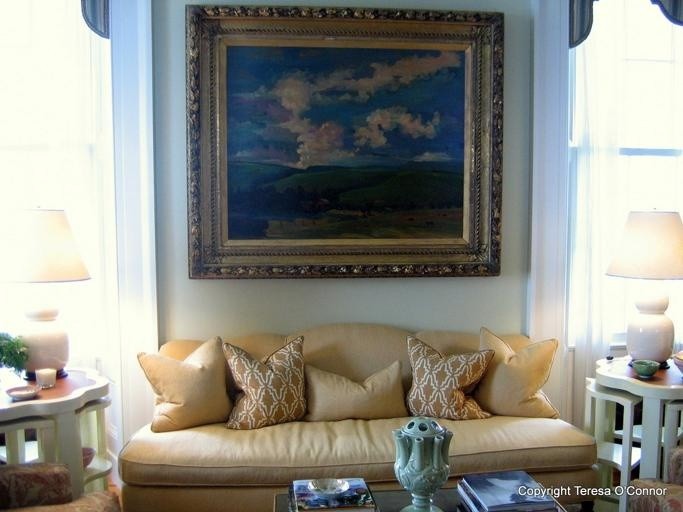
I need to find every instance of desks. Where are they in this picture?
[584,355,683,512]
[1,369,113,498]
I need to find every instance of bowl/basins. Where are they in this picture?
[82,448,96,469]
[631,359,660,379]
[308,478,350,500]
[672,350,682,380]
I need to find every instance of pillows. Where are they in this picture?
[138,334,232,432]
[306,360,408,422]
[477,329,561,418]
[221,337,306,429]
[406,335,495,419]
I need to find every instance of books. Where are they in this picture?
[456,470,561,512]
[290,476,377,512]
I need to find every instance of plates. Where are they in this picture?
[5,386,40,400]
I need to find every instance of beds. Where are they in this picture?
[273,490,635,511]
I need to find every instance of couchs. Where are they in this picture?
[627,438,683,512]
[118,323,598,511]
[1,461,125,509]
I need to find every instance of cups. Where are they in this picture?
[34,368,57,389]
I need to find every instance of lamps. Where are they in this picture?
[0,208,99,375]
[604,211,683,369]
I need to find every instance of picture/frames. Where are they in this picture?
[184,4,503,277]
